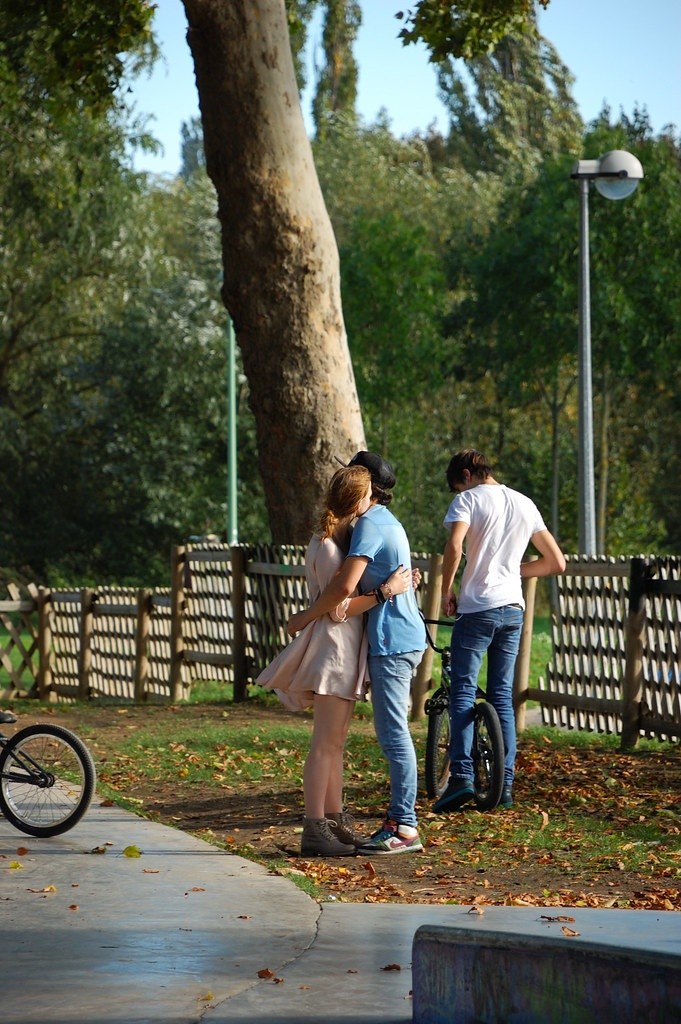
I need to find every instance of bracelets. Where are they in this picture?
[373,587,387,606]
[381,583,394,607]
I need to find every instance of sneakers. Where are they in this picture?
[358,820,422,854]
[433,779,475,813]
[499,786,513,807]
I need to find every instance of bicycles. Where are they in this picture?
[3,712,95,834]
[414,604,506,814]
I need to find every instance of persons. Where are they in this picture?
[430,451,566,813]
[288,451,426,856]
[252,465,425,859]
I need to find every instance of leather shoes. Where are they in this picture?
[325,813,373,848]
[301,816,356,855]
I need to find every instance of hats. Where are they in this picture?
[348,452,396,489]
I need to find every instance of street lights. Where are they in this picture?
[574,144,642,554]
